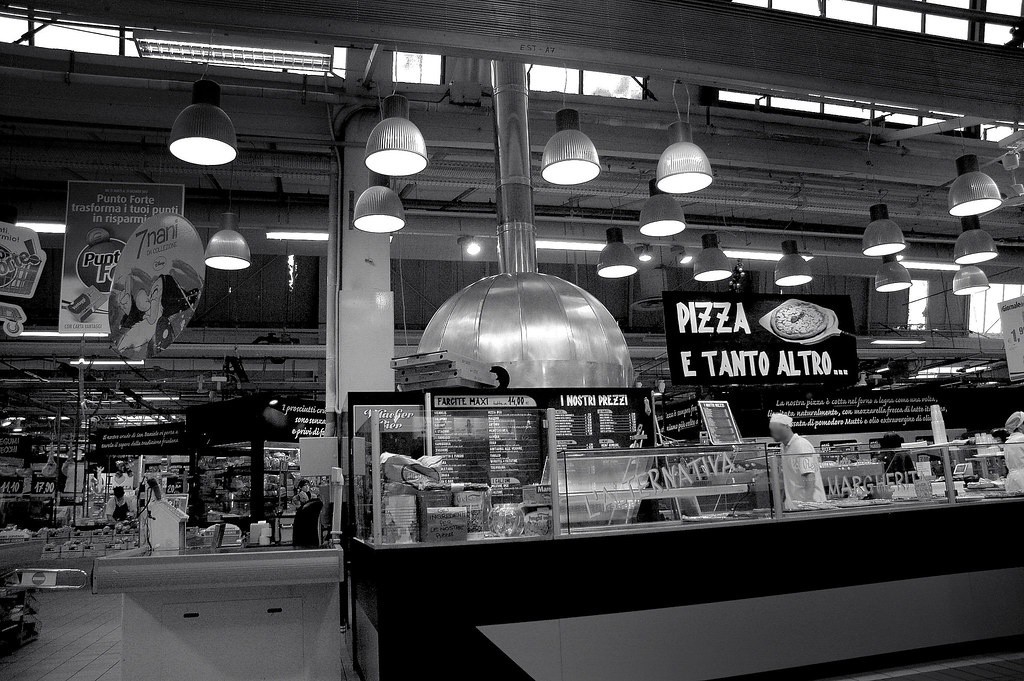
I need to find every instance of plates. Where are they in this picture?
[956,496,984,501]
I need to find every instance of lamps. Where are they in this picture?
[132,26,480,270]
[542,66,1003,295]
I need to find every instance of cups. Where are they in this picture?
[915,481,933,502]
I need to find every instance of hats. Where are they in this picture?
[113,486,124,497]
[770,413,793,428]
[1005,411,1024,433]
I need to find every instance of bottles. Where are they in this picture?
[487,503,551,535]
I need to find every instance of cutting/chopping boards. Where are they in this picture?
[758,299,843,345]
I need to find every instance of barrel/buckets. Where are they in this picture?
[382,492,418,543]
[453,488,485,540]
[419,486,451,542]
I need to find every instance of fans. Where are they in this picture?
[978,150,1024,220]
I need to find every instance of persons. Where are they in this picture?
[293,480,317,507]
[106,486,133,524]
[769,413,825,504]
[878,432,919,484]
[1005,411,1024,494]
